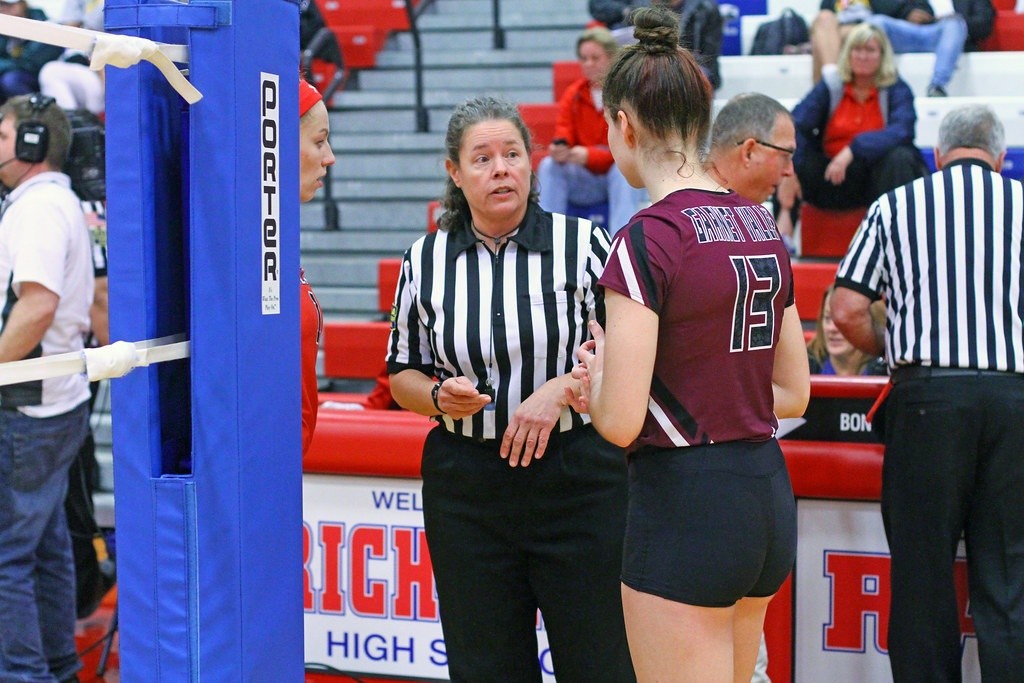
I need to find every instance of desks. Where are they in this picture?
[302,409,981,683]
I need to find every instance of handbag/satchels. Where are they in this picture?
[749,8,809,55]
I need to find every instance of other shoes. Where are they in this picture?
[927,84,948,97]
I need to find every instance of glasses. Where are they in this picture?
[736,140,795,159]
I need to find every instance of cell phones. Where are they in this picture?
[555,138,567,146]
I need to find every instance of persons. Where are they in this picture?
[564,7,811,683]
[534,27,650,242]
[806,284,889,376]
[812,0,872,88]
[389,95,636,683]
[0,0,106,683]
[705,95,797,683]
[589,0,722,93]
[773,20,925,258]
[829,105,1024,683]
[300,76,336,465]
[865,0,995,97]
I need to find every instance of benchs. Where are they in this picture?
[74,0,1024,445]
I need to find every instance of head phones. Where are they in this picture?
[15,93,55,163]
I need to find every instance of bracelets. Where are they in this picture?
[432,383,447,414]
